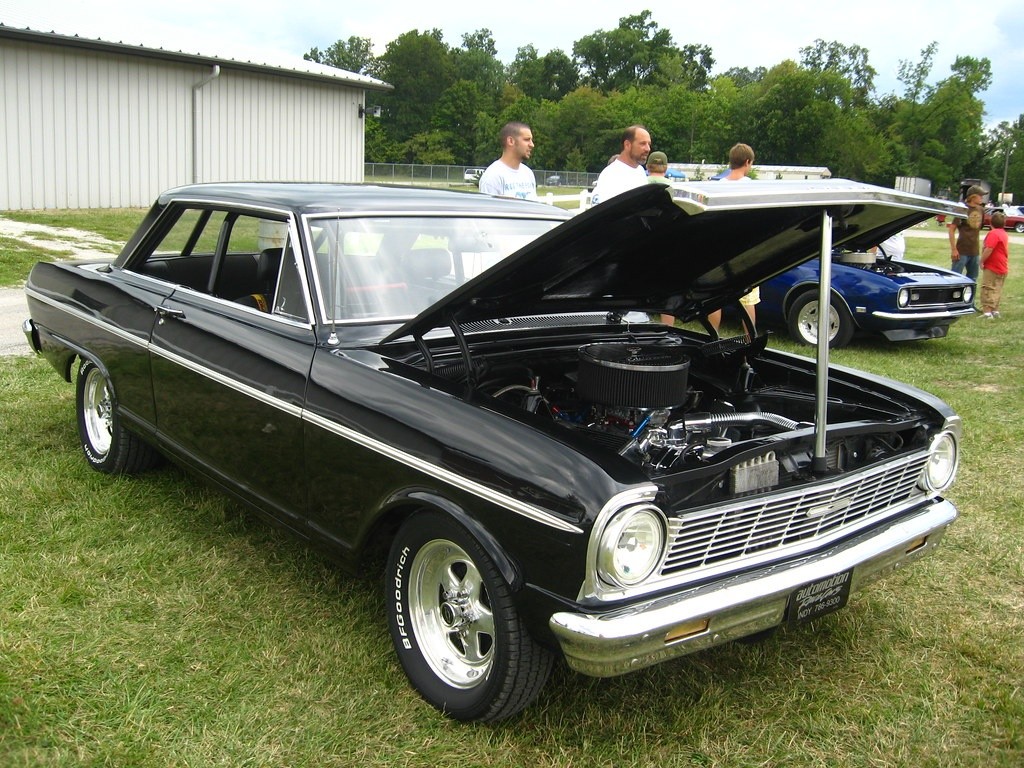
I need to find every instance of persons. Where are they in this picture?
[949,185,989,313]
[646,150,675,327]
[590,125,652,209]
[478,120,537,202]
[707,142,761,338]
[875,232,906,261]
[976,210,1009,319]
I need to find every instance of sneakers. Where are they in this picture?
[991,312,1001,319]
[974,314,994,322]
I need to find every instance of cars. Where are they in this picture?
[464,168,486,182]
[980,205,1024,234]
[20,179,971,723]
[716,242,980,349]
[546,176,570,186]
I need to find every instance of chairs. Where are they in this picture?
[247,247,305,314]
[405,249,457,309]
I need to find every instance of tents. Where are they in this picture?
[709,167,732,180]
[645,167,687,182]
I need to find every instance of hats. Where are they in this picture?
[645,151,668,166]
[966,186,989,197]
